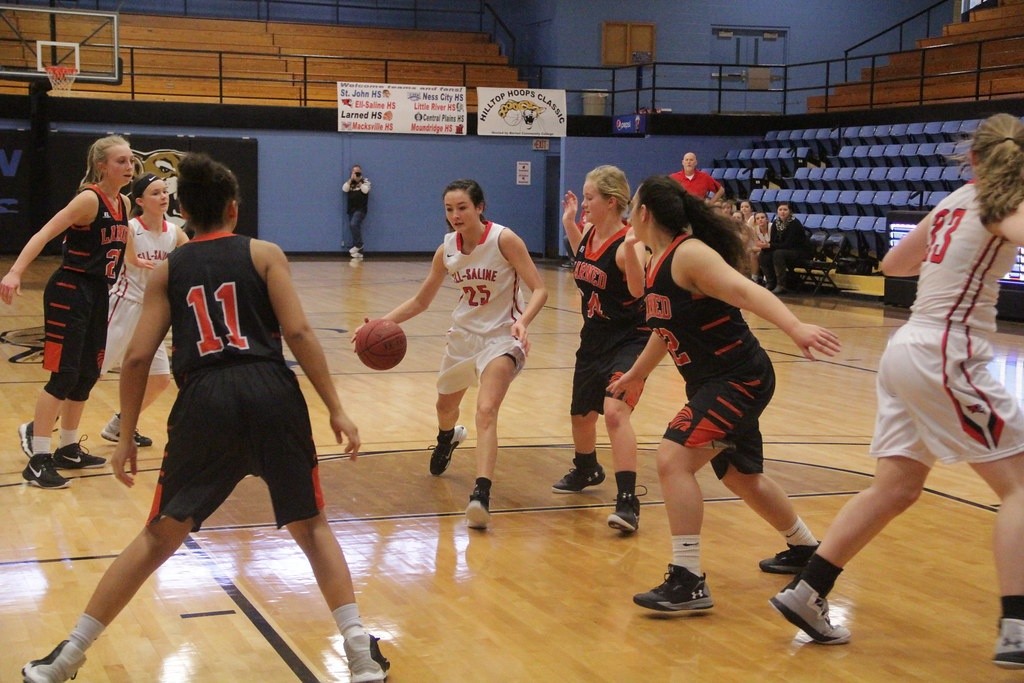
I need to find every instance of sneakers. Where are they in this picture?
[465,485,491,528]
[634,563,714,611]
[341,633,390,683]
[428,424,466,475]
[607,483,647,531]
[552,463,606,493]
[20,639,87,683]
[769,576,852,644]
[101,412,152,447]
[19,418,60,457]
[53,435,107,469]
[759,540,824,574]
[22,452,70,489]
[991,616,1024,668]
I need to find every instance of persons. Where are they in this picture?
[768,115,1024,669]
[349,179,548,527]
[705,198,732,216]
[0,133,156,487]
[605,174,842,611]
[732,199,773,286]
[19,172,190,447]
[669,151,725,207]
[341,165,371,257]
[21,153,392,683]
[552,165,645,532]
[758,202,814,294]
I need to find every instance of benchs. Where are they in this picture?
[807,0,1024,112]
[0,7,528,97]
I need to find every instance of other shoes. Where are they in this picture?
[765,282,777,290]
[772,284,790,294]
[350,253,363,258]
[349,244,365,254]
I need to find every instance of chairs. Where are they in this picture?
[799,232,848,296]
[791,230,830,290]
[700,119,988,255]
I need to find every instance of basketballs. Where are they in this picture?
[355,318,408,371]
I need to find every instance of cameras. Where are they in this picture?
[355,172,361,177]
